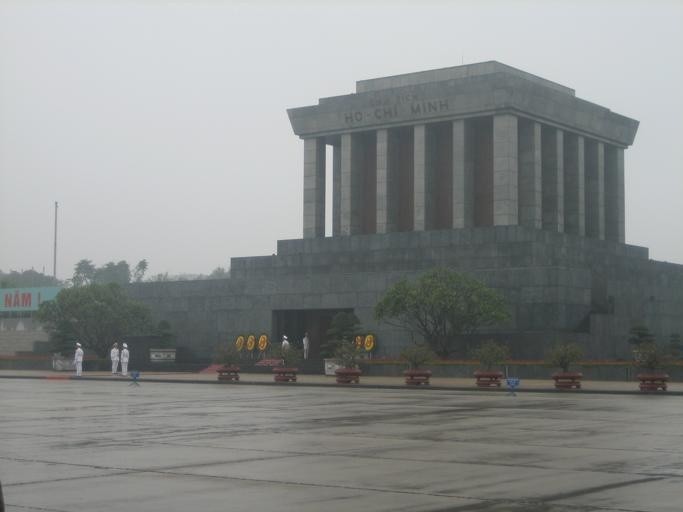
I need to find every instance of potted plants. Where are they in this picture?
[544,342,585,388]
[635,342,669,390]
[471,340,513,387]
[272,346,299,381]
[334,344,362,385]
[216,341,240,381]
[402,346,432,385]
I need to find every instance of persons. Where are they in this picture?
[119,343,129,376]
[72,341,84,377]
[301,331,310,361]
[108,342,120,374]
[280,334,289,354]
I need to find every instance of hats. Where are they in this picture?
[75,342,81,348]
[282,335,288,339]
[122,343,128,348]
